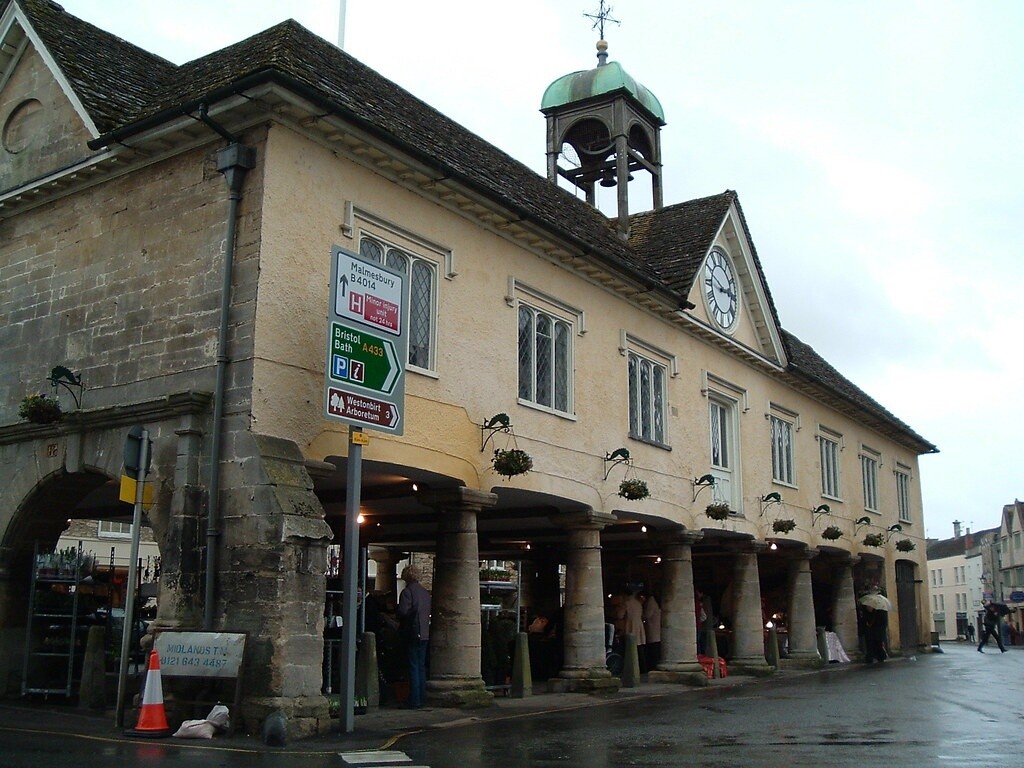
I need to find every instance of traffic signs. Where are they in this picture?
[322,240,410,437]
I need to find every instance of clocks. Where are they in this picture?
[698,243,743,336]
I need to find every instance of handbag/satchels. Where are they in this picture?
[399,588,421,647]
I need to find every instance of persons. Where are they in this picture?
[611,589,659,674]
[397,565,431,710]
[978,605,1008,653]
[864,609,887,663]
[1002,620,1017,646]
[490,610,518,684]
[324,581,406,686]
[694,586,716,657]
[967,623,975,643]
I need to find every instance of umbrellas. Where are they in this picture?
[857,594,892,611]
[983,602,1010,617]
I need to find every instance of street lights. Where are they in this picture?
[980,574,1001,641]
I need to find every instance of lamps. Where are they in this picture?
[979,574,992,584]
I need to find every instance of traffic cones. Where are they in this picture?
[133,649,170,731]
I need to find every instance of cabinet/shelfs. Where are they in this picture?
[324,546,366,695]
[20,539,116,715]
[480,560,522,698]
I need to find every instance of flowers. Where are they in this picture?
[17,392,61,421]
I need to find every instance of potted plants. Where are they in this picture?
[33,547,94,580]
[863,533,886,547]
[478,567,514,582]
[895,537,917,553]
[617,479,651,502]
[378,637,410,703]
[481,612,499,692]
[821,526,843,541]
[706,502,730,521]
[773,518,797,534]
[490,618,518,685]
[490,447,534,483]
